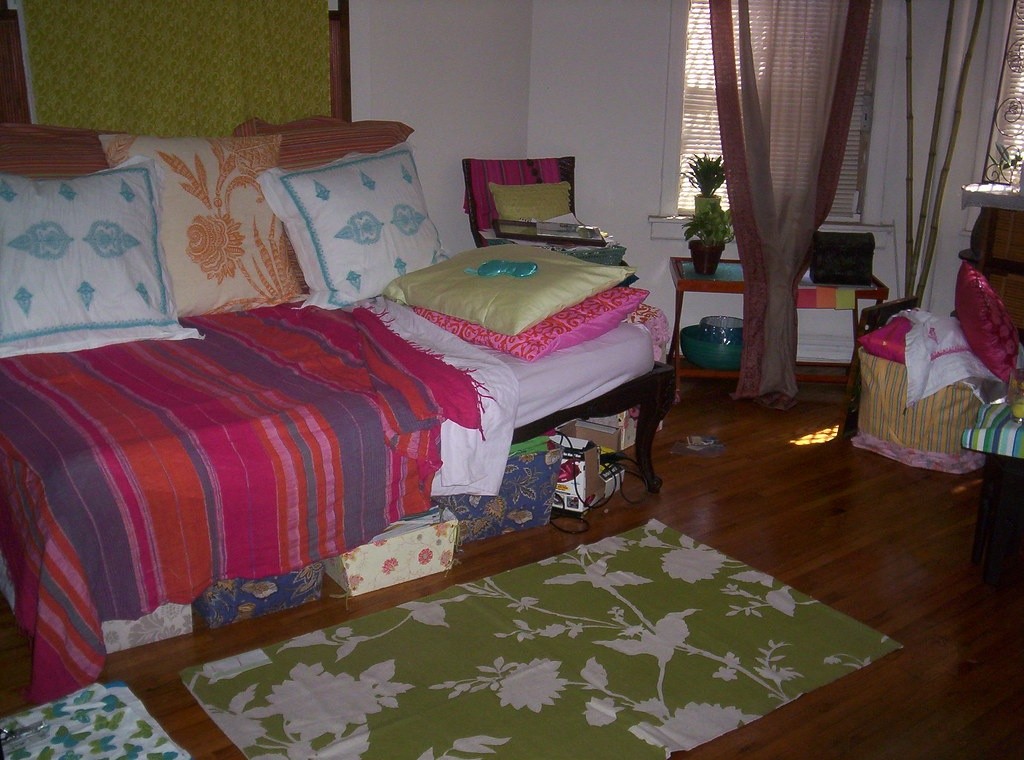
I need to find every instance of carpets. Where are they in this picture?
[178,518,903,760]
[0,681,195,760]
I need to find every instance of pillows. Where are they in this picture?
[385,245,635,337]
[97,134,308,320]
[415,285,649,360]
[0,157,202,362]
[233,117,412,175]
[955,259,1018,381]
[488,181,571,232]
[256,143,452,312]
[856,310,911,365]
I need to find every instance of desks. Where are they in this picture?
[963,394,1024,588]
[668,256,889,400]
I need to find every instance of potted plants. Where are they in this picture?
[683,209,737,276]
[682,154,728,216]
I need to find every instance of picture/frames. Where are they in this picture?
[492,218,607,247]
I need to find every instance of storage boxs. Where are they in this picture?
[810,232,875,286]
[101,604,194,656]
[433,438,566,544]
[324,508,458,598]
[194,558,326,629]
[855,347,982,470]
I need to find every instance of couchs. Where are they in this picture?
[0,127,677,706]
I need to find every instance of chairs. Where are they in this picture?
[462,155,636,286]
